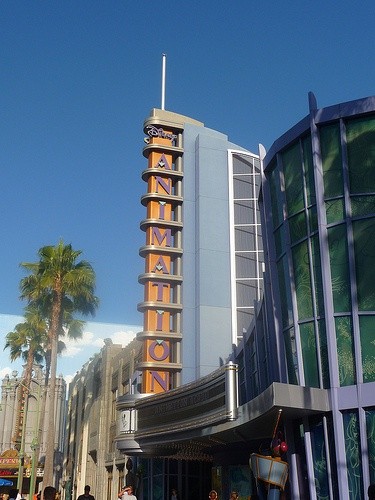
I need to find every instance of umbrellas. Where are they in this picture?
[0,479,13,486]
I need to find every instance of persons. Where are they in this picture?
[230,492,238,500]
[43,486,56,500]
[118,486,137,500]
[172,489,178,500]
[77,486,95,500]
[209,490,218,500]
